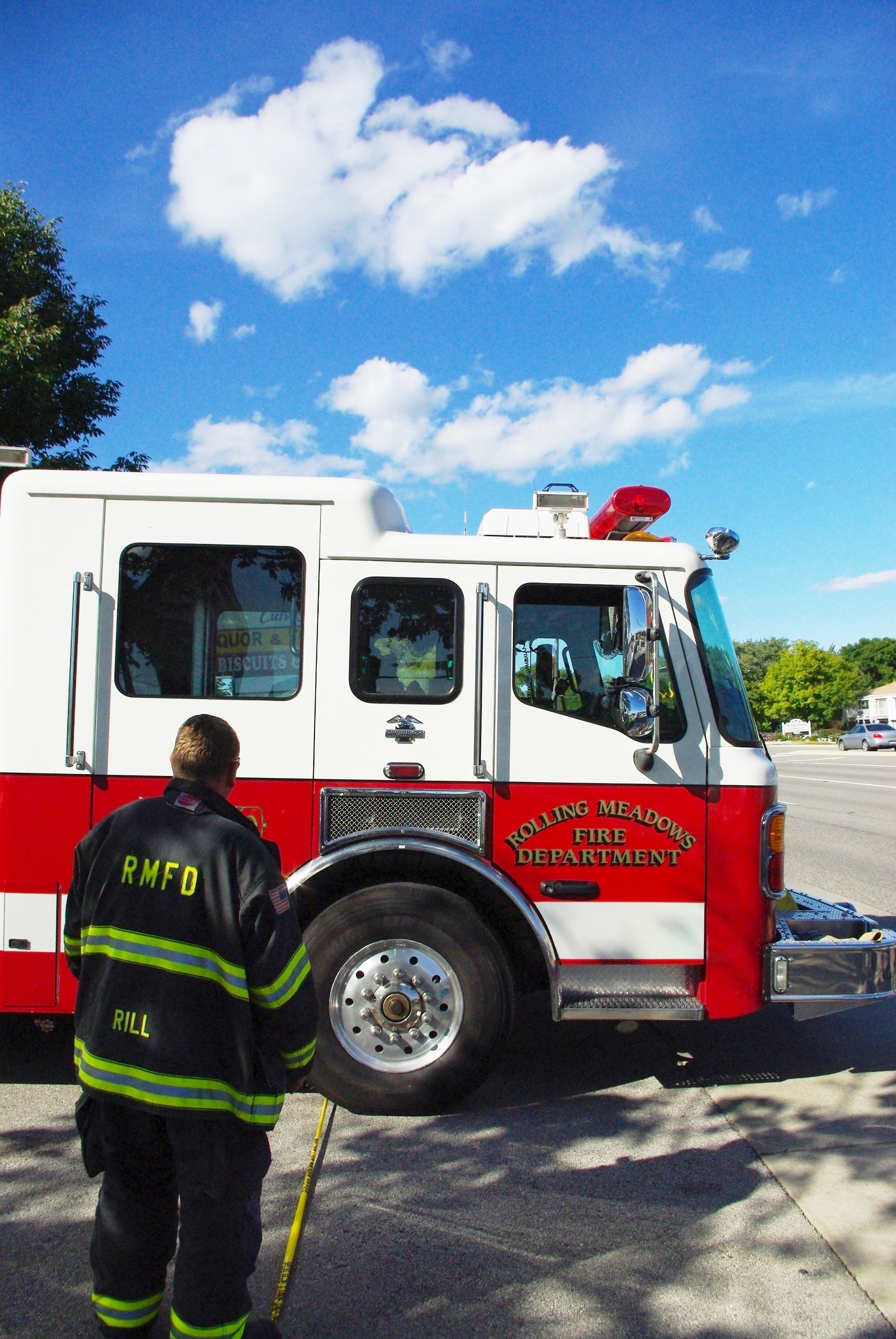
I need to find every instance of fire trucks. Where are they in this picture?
[0,446,896,1118]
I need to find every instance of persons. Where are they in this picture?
[61,712,321,1339]
[370,608,455,696]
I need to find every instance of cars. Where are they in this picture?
[800,734,819,739]
[837,723,896,752]
[761,734,773,741]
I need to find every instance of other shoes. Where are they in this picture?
[240,1318,284,1339]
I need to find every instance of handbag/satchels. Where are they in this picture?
[75,1091,104,1178]
